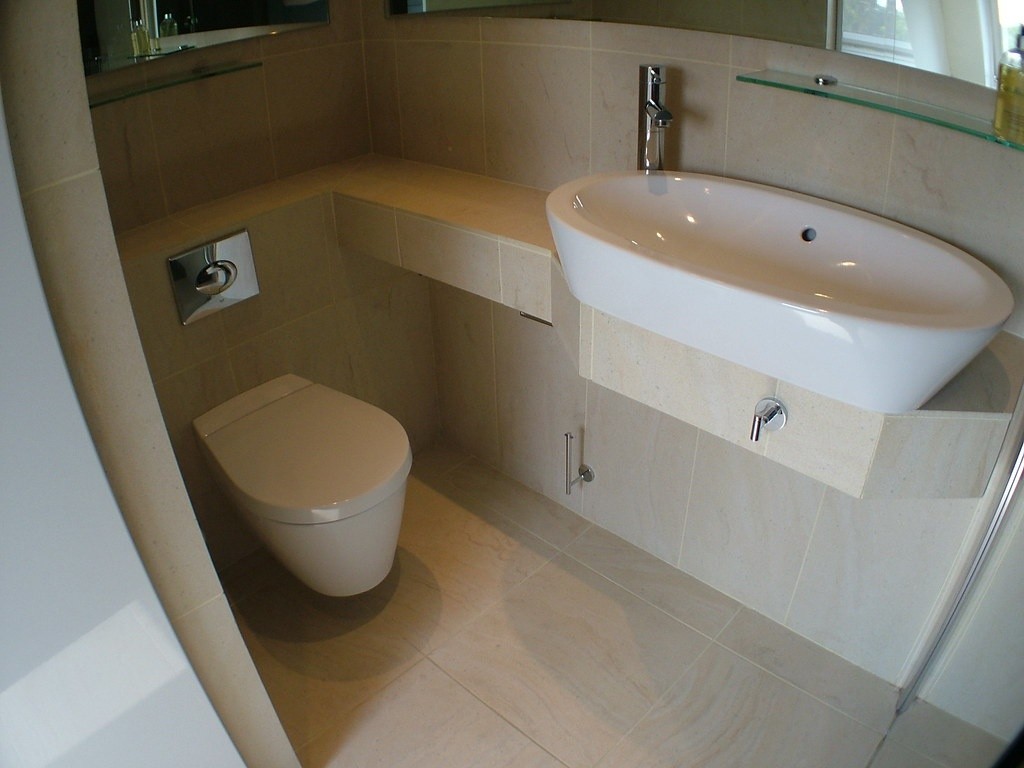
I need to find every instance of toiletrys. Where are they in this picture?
[992,24,1024,144]
[124,14,150,54]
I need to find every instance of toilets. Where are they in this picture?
[190,371,413,598]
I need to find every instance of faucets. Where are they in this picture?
[638,62,672,172]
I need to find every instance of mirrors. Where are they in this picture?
[387,0,1024,89]
[76,0,331,77]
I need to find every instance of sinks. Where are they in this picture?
[541,163,1015,412]
